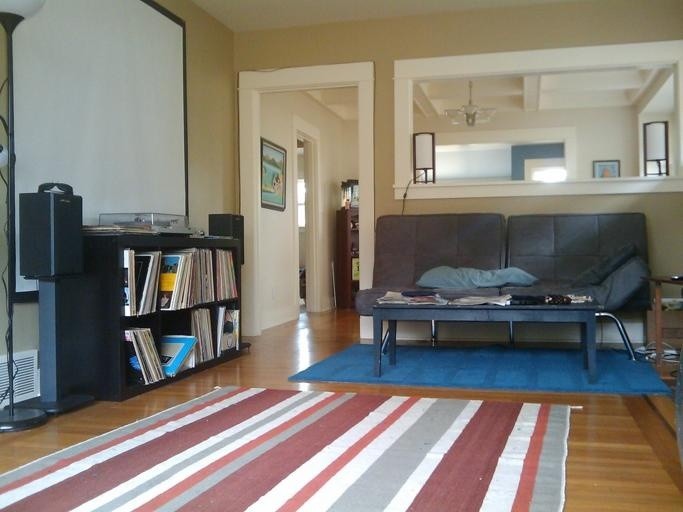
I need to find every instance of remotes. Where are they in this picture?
[670,275,683,281]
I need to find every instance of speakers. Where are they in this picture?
[17,191,84,277]
[208,212,244,266]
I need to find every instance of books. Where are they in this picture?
[83,223,155,235]
[121,246,240,386]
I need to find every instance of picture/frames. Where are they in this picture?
[260,136,287,212]
[592,160,620,178]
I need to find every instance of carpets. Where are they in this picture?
[0,385,571,512]
[289,344,672,395]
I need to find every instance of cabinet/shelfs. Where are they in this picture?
[336,208,359,311]
[55,237,251,403]
[640,276,683,378]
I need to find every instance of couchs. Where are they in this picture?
[355,213,653,361]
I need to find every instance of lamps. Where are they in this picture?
[444,80,496,127]
[642,121,670,177]
[413,132,435,184]
